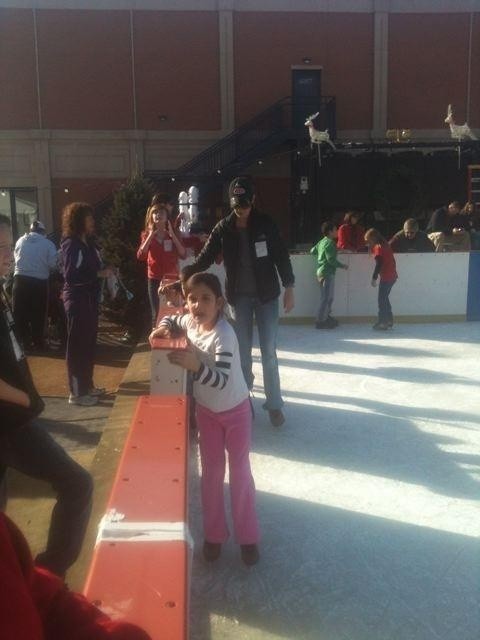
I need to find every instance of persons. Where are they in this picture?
[145,192,184,239]
[364,228,399,330]
[149,272,260,565]
[460,200,480,233]
[426,201,471,252]
[0,512,152,640]
[310,221,349,329]
[137,204,187,331]
[12,221,58,350]
[180,176,296,428]
[61,202,111,406]
[336,211,366,251]
[0,212,93,582]
[389,217,435,252]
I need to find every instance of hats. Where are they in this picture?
[228,176,255,209]
[29,221,45,230]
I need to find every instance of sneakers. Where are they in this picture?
[314,316,339,328]
[68,393,100,406]
[240,543,260,564]
[269,408,285,427]
[372,320,387,330]
[87,387,106,396]
[388,319,394,329]
[203,531,228,561]
